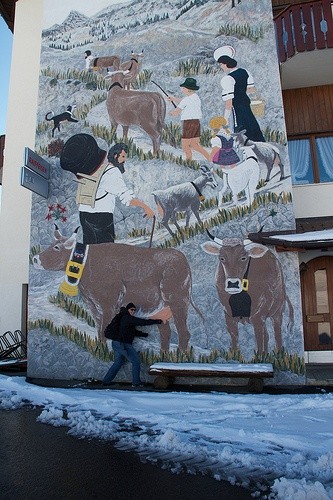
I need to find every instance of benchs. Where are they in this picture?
[146,362,275,393]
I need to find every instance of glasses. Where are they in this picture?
[130,308,136,311]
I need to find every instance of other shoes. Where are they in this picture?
[132,384,144,388]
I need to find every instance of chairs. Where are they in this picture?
[0,330,28,361]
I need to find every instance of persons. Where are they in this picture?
[101,302,162,388]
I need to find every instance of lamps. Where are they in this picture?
[299,262,308,272]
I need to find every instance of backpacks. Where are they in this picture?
[104,309,127,346]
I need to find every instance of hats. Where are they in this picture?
[179,78,199,91]
[126,303,136,310]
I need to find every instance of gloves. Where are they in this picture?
[140,333,148,338]
[152,319,162,325]
